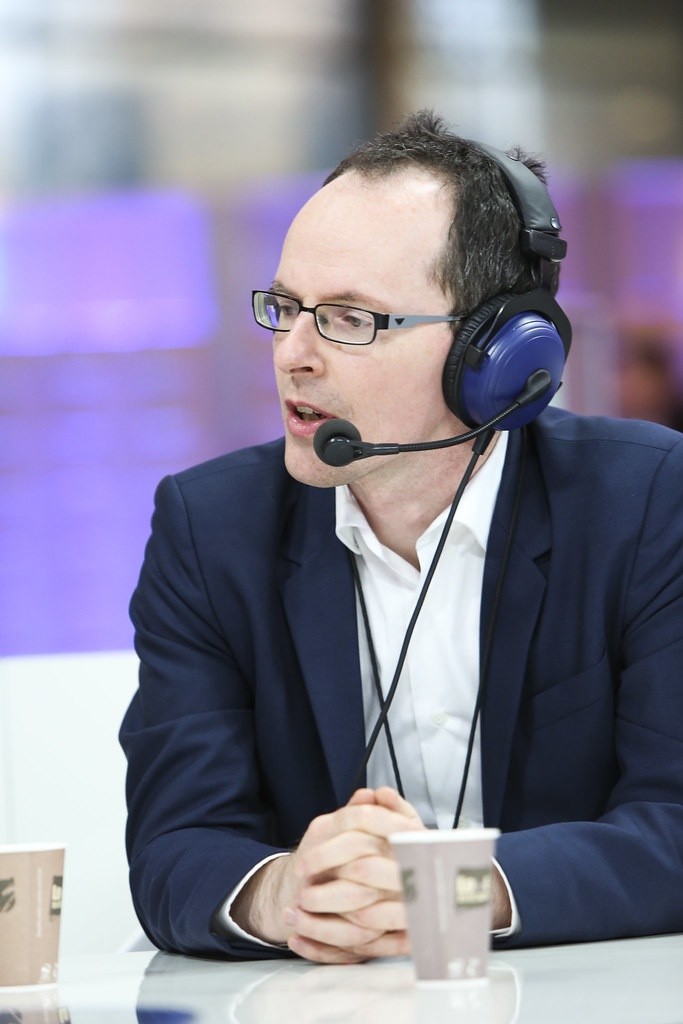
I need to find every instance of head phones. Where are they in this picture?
[442,141,573,456]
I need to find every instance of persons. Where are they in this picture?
[120,112,682,964]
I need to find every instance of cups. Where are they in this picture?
[0,844,68,992]
[388,828,503,988]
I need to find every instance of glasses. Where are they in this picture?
[249,287,469,346]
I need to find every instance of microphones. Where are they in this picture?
[312,402,520,467]
